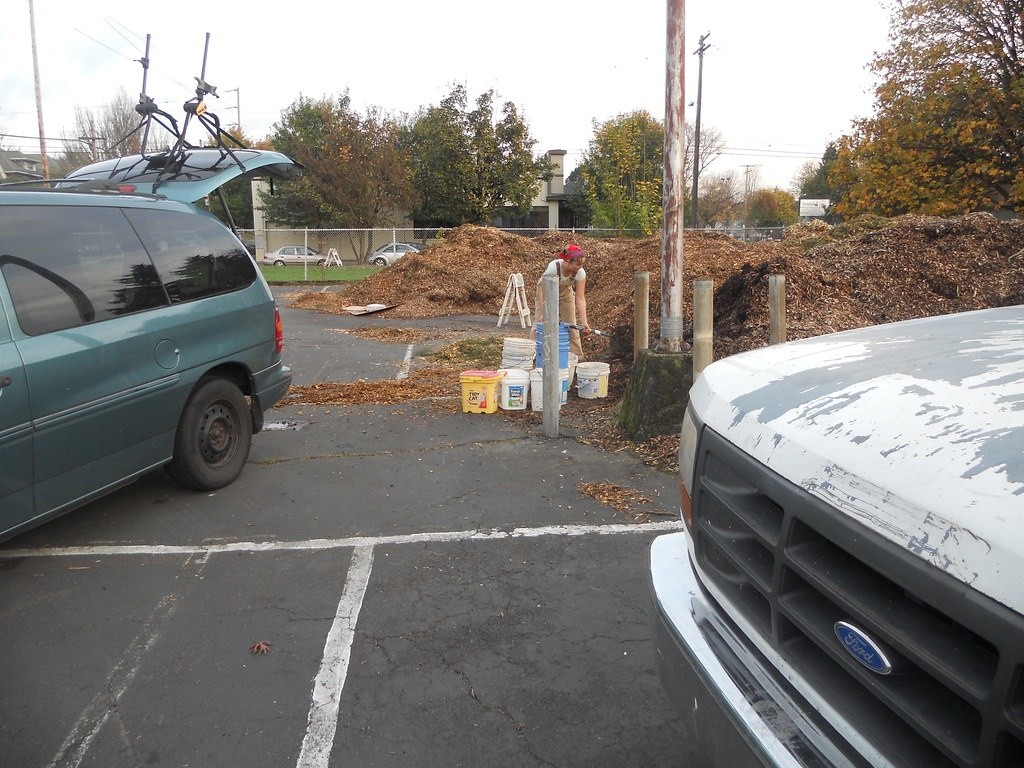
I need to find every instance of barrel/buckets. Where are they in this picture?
[459,370,508,414]
[574,362,610,400]
[568,352,578,386]
[497,368,530,410]
[536,322,570,410]
[530,368,543,412]
[499,337,537,370]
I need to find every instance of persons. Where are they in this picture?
[528,242,592,356]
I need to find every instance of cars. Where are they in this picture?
[366,241,429,267]
[262,244,328,266]
[644,304,1024,768]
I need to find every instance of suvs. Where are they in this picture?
[0,32,308,544]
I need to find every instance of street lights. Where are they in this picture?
[720,177,730,221]
